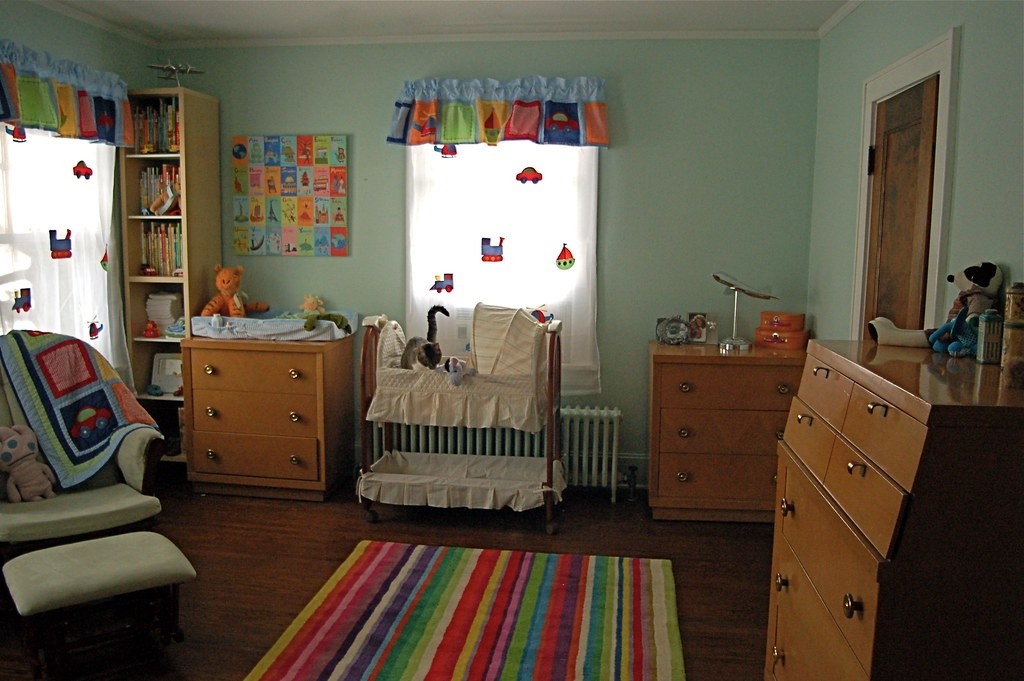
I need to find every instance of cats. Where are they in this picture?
[400,305,450,371]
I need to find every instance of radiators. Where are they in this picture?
[371,401,620,505]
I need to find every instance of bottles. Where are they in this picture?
[212,314,223,328]
[224,321,233,327]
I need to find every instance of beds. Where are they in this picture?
[356,315,567,535]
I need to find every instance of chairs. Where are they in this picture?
[0,329,168,565]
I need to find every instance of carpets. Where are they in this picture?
[243,541,688,681]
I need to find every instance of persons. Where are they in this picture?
[687,314,706,342]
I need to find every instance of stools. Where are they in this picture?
[2,531,196,681]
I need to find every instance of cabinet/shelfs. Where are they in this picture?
[765,341,1024,681]
[119,87,221,462]
[647,340,807,525]
[181,334,354,503]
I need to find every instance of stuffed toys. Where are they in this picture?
[297,294,353,335]
[0,423,56,502]
[199,264,270,322]
[436,356,476,386]
[867,262,1003,350]
[928,306,979,357]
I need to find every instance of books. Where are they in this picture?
[131,95,179,340]
